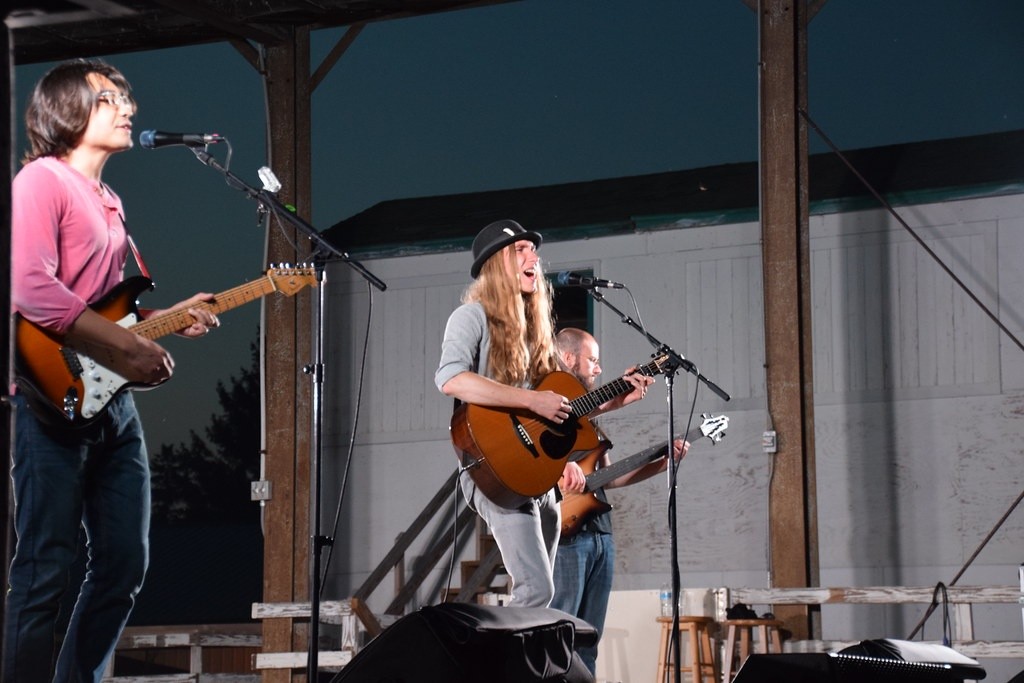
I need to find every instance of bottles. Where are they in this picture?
[660,581,672,616]
[712,585,729,622]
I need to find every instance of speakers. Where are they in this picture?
[325,601,597,683]
[731,635,988,683]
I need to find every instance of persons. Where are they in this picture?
[549,328,691,682]
[434,218,656,608]
[0,57,220,683]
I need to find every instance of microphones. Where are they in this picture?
[342,255,388,295]
[138,128,225,151]
[689,368,730,404]
[558,270,625,290]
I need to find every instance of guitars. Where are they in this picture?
[450,348,679,509]
[560,412,731,544]
[10,261,328,432]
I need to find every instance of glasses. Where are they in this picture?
[96,91,133,108]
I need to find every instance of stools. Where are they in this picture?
[723,617,784,683]
[656,615,720,683]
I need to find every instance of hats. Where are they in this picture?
[470,220,543,281]
